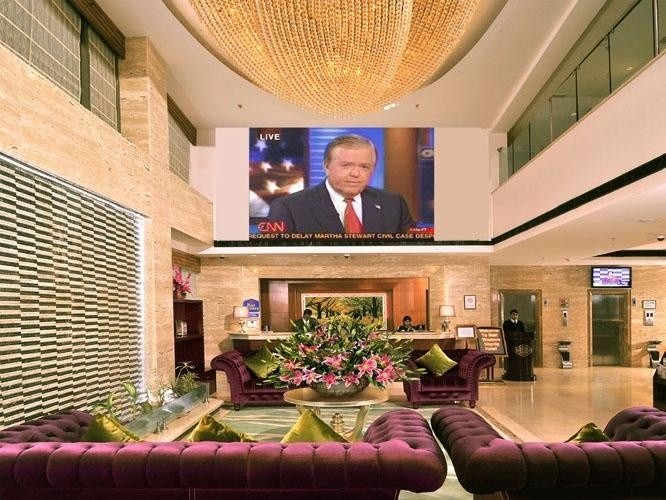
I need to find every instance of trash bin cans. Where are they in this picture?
[646,341,662,368]
[557,341,572,369]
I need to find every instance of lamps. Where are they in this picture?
[162,0,509,118]
[233,307,249,333]
[439,305,456,332]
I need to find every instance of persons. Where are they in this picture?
[295,309,319,332]
[267,134,414,235]
[396,316,417,332]
[503,308,525,330]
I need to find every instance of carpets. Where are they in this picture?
[179,401,526,500]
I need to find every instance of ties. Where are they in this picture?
[514,320,517,324]
[342,198,364,234]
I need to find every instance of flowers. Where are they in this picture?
[256,309,427,389]
[173,264,195,297]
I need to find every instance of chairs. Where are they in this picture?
[403,348,496,409]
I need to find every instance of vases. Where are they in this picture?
[175,292,188,299]
[308,380,369,398]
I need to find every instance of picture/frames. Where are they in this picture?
[464,294,476,309]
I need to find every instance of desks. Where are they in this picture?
[283,385,388,443]
[146,397,225,441]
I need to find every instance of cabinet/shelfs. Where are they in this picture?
[173,299,206,385]
[205,366,216,394]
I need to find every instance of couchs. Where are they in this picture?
[0,407,446,500]
[431,407,666,500]
[210,350,295,410]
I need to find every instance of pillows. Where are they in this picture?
[243,345,282,379]
[281,409,355,442]
[414,344,457,377]
[562,422,611,443]
[185,415,259,443]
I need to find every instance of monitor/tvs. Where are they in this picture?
[589,266,633,289]
[247,126,436,241]
